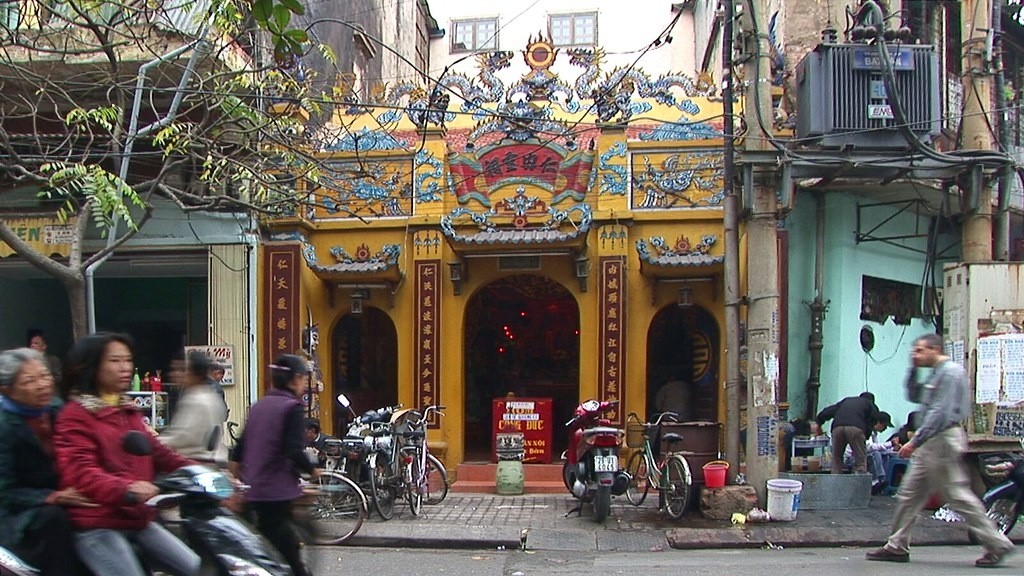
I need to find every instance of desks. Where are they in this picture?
[792,438,829,459]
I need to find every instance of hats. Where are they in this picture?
[272,354,310,376]
[878,412,894,428]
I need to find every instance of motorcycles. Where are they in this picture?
[966,431,1024,547]
[560,398,634,524]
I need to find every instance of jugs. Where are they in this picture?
[790,456,804,472]
[808,456,821,472]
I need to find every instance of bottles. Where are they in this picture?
[144,374,151,391]
[132,368,140,391]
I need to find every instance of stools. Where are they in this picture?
[882,458,910,495]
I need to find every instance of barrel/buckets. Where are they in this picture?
[765,480,803,520]
[659,421,720,512]
[496,460,525,495]
[702,461,729,487]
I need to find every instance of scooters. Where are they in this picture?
[0,425,296,576]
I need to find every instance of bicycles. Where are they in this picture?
[287,393,449,546]
[625,410,693,520]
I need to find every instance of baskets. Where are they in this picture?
[979,451,1022,486]
[396,420,428,446]
[626,422,659,448]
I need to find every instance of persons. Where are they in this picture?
[866,333,1016,567]
[227,354,315,576]
[652,376,698,421]
[53,332,200,576]
[156,349,230,547]
[300,417,337,469]
[29,335,63,398]
[843,411,895,495]
[813,392,879,474]
[885,411,921,446]
[0,347,99,576]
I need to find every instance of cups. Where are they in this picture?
[152,376,161,391]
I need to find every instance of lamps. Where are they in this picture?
[576,256,590,291]
[352,281,365,315]
[448,260,462,295]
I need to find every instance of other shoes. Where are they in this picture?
[975,547,1014,567]
[867,547,910,561]
[881,479,890,487]
[870,481,885,496]
